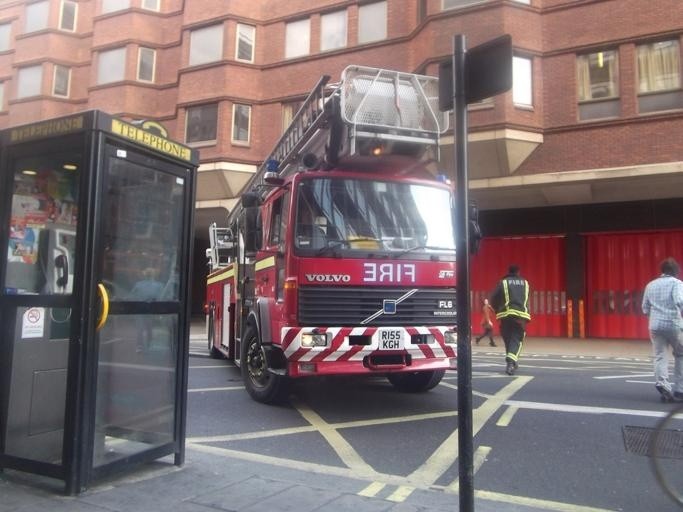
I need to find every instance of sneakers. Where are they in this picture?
[506,364,513,375]
[661,393,683,403]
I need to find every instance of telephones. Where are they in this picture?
[34,224,77,342]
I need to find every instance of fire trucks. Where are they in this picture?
[204,64,484,405]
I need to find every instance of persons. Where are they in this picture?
[492,263,532,377]
[473,295,497,348]
[122,266,164,356]
[640,258,682,403]
